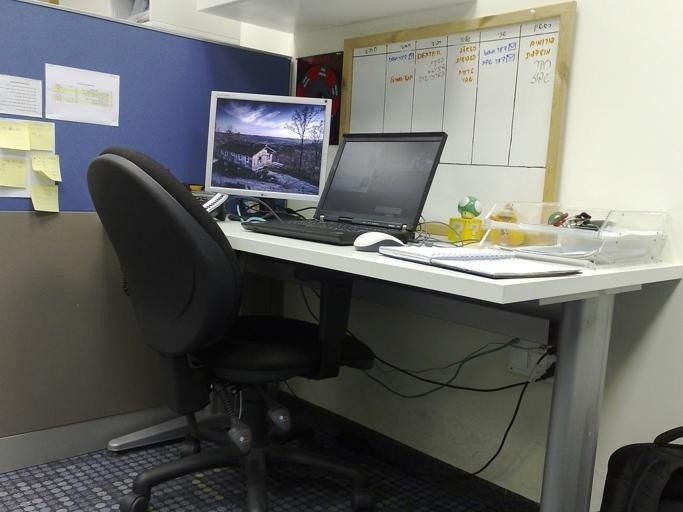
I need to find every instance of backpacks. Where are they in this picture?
[600,426,683,512]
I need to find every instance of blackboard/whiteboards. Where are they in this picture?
[339,0,577,224]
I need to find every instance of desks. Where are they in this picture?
[212,216,683,509]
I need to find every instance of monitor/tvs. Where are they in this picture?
[203,90,332,219]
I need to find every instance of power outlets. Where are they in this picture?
[544,354,557,385]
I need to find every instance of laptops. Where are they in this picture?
[240,131,448,246]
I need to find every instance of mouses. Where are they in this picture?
[354,231,404,252]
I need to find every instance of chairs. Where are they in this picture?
[88,148,377,510]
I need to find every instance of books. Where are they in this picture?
[378,242,583,281]
